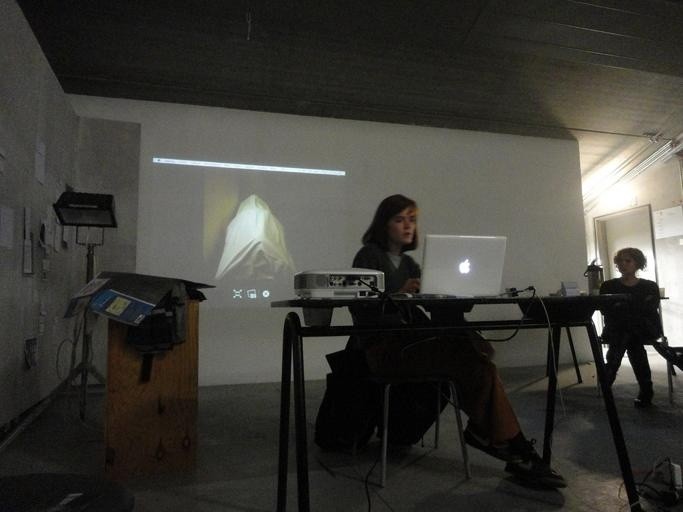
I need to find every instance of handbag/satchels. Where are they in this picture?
[616,457,682,510]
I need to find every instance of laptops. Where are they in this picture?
[411,232,510,299]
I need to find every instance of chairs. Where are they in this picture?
[597,310,676,404]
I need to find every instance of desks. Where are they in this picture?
[269,292,644,512]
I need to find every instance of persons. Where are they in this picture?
[598,247,662,405]
[343,192,568,492]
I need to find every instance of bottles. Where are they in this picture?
[584,260,604,297]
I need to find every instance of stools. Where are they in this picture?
[382,371,473,487]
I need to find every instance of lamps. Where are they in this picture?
[51,191,117,421]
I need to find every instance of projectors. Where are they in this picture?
[293,269,387,302]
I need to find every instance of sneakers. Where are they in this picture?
[635,387,653,409]
[464,423,566,489]
